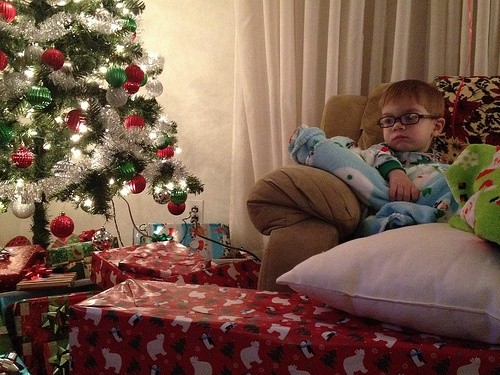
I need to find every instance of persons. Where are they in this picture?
[360,79,446,203]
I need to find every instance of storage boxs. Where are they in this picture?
[0,244,500,375]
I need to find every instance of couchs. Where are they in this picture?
[245,76,500,291]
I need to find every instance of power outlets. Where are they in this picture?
[188,199,204,224]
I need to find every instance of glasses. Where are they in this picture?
[377,113,440,128]
